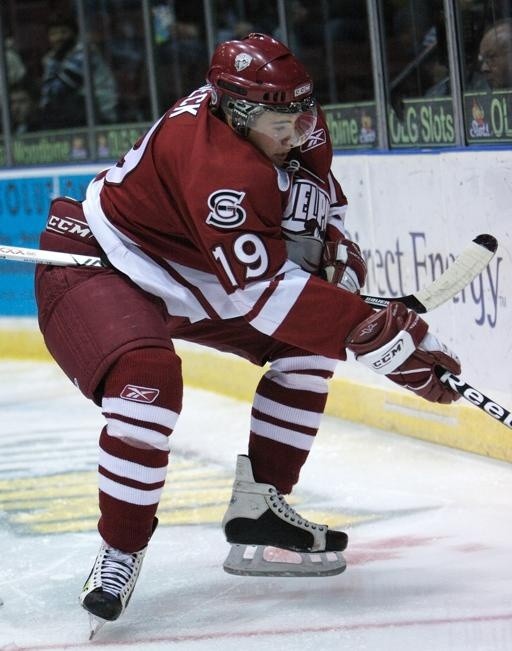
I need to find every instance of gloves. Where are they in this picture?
[343,302,462,405]
[321,238,368,294]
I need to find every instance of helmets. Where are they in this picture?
[203,32,317,150]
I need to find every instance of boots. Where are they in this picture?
[77,517,159,622]
[220,456,348,553]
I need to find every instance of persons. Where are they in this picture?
[34,31,464,641]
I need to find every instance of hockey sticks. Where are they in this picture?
[0,235,497,313]
